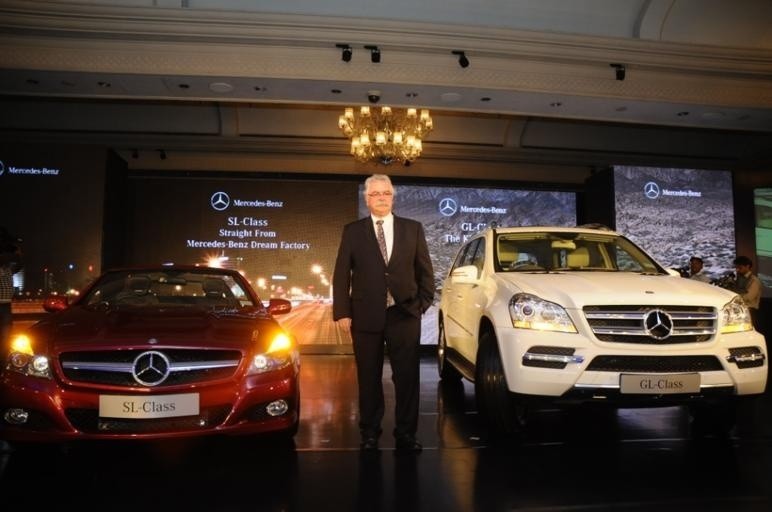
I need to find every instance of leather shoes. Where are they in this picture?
[360,428,382,451]
[396,440,423,450]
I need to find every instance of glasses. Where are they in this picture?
[368,191,392,197]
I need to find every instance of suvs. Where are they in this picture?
[437,225,770,412]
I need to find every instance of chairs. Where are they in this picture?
[565,246,590,270]
[498,243,519,271]
[193,277,234,311]
[116,275,161,313]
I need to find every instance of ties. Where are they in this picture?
[376,220,392,307]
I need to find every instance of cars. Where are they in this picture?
[0,263,301,452]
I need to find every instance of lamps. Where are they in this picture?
[336,43,353,62]
[451,50,469,68]
[364,45,381,63]
[609,63,625,81]
[337,106,434,167]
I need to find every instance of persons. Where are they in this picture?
[0,243,25,324]
[734,256,762,327]
[690,257,709,283]
[332,175,434,453]
